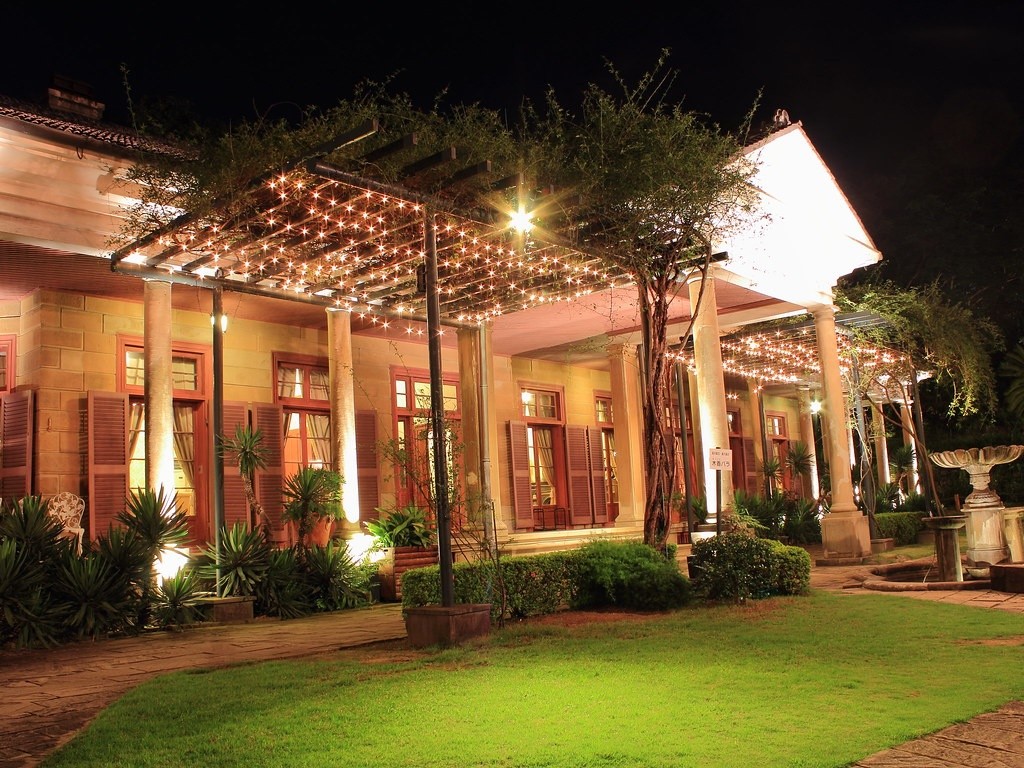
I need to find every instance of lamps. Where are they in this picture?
[810,389,824,413]
[210,310,228,334]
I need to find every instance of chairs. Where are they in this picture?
[11,490,86,557]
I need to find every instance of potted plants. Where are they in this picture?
[362,493,439,603]
[673,492,756,545]
[281,464,347,548]
